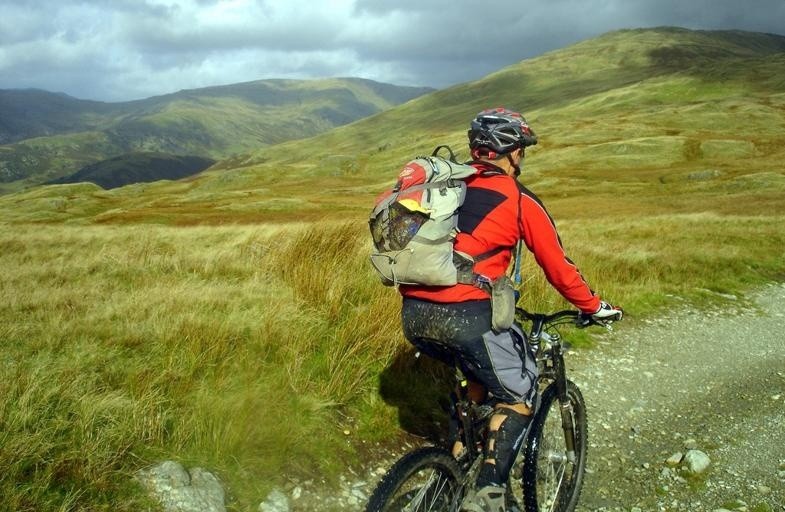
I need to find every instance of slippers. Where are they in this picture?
[367,144,493,291]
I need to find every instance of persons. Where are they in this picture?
[396,106,625,512]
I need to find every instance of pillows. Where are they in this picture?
[468,108,538,159]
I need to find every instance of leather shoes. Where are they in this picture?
[590,301,623,327]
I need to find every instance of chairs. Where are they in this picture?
[462,482,507,512]
